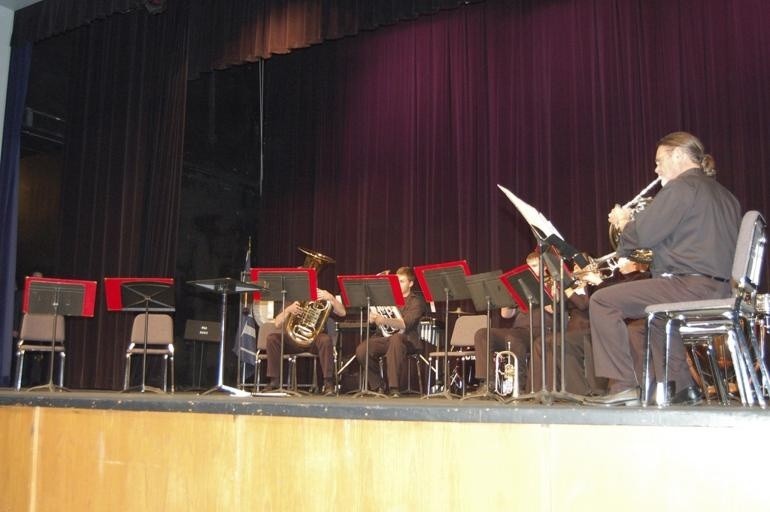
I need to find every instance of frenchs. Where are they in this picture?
[608,178,661,264]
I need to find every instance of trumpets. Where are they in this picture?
[574,252,620,278]
[494,342,518,402]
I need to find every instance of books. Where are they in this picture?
[497,184,567,241]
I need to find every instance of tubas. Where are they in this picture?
[370,270,405,337]
[284,248,336,347]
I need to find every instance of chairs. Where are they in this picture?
[121,311,176,395]
[12,314,68,392]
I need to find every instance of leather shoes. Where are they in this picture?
[261,386,401,398]
[583,389,640,407]
[670,386,703,407]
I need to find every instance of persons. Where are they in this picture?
[355,266,425,399]
[473,251,560,401]
[581,132,743,406]
[523,251,651,407]
[259,266,347,397]
